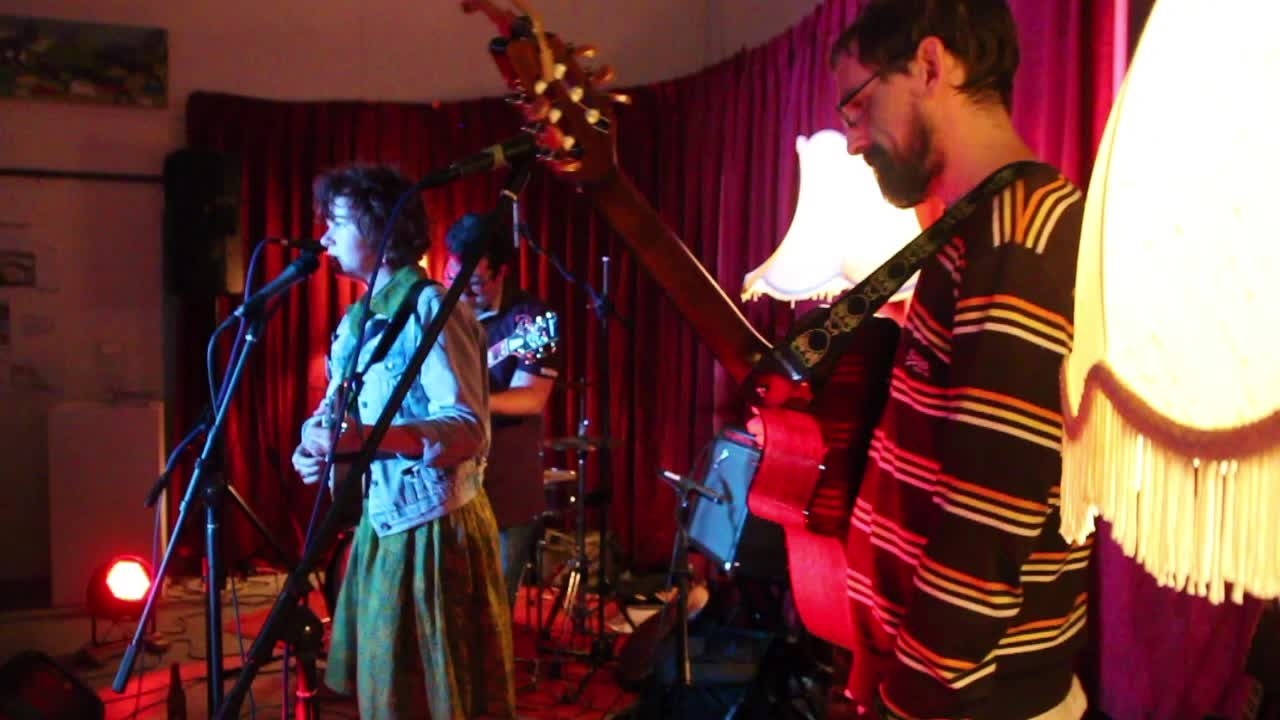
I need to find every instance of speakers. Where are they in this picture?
[686,425,781,575]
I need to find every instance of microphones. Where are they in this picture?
[417,135,535,190]
[657,468,725,505]
[514,199,520,248]
[271,238,326,252]
[224,251,320,328]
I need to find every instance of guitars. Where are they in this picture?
[329,316,390,523]
[483,304,556,369]
[487,14,904,654]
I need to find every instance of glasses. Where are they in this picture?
[834,60,897,127]
[444,269,497,289]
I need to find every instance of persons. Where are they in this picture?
[291,166,514,720]
[832,0,1086,719]
[447,212,561,614]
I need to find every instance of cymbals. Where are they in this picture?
[544,469,577,484]
[541,436,596,452]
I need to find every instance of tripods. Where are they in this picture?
[520,222,634,703]
